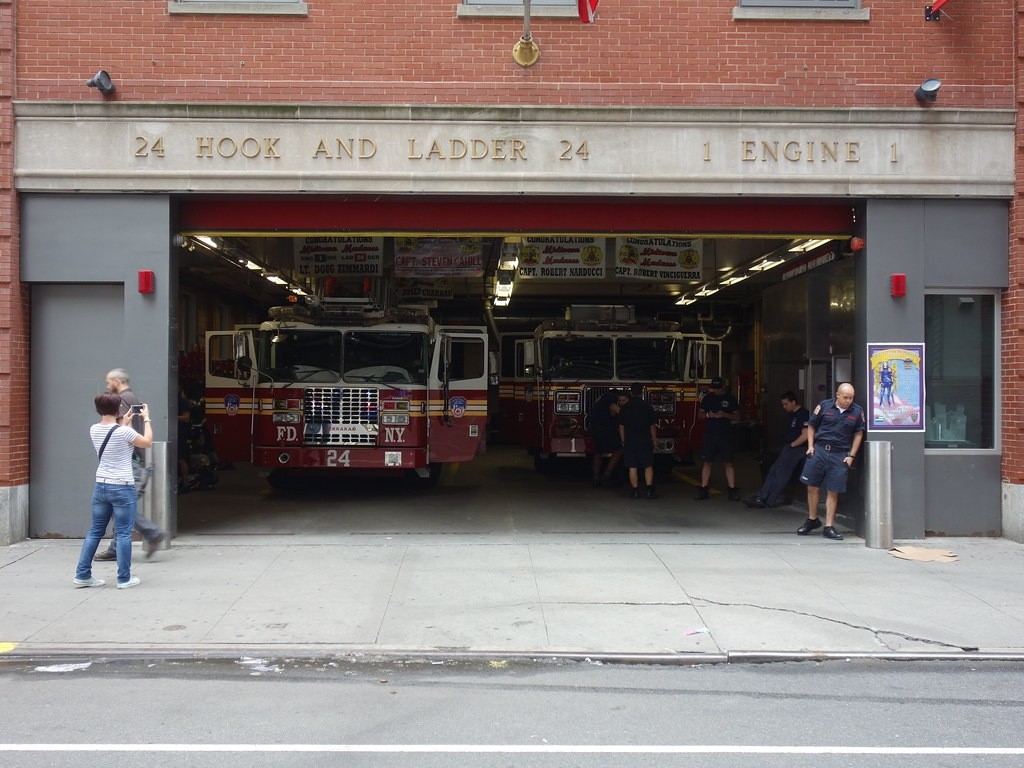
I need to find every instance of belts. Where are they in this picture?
[96,477,134,487]
[816,440,849,452]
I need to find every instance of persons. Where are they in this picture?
[177,387,192,494]
[73,391,153,589]
[590,383,659,499]
[695,375,742,501]
[796,383,866,540]
[742,391,812,507]
[93,368,164,562]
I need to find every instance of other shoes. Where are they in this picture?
[645,485,657,498]
[630,487,640,499]
[695,485,708,500]
[728,486,740,501]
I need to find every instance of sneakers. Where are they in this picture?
[147,532,165,558]
[118,578,140,589]
[823,527,843,540]
[94,548,117,561]
[796,516,822,535]
[73,576,106,587]
[743,495,774,508]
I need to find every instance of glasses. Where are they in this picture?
[120,403,123,411]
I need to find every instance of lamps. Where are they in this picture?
[86,70,115,95]
[177,235,834,307]
[913,79,942,100]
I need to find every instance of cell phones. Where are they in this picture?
[131,405,144,414]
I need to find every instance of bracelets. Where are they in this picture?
[705,412,709,419]
[144,419,151,422]
[849,455,855,458]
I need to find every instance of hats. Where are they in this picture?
[711,377,722,387]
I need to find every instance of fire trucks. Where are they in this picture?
[205,270,489,492]
[497,304,723,478]
